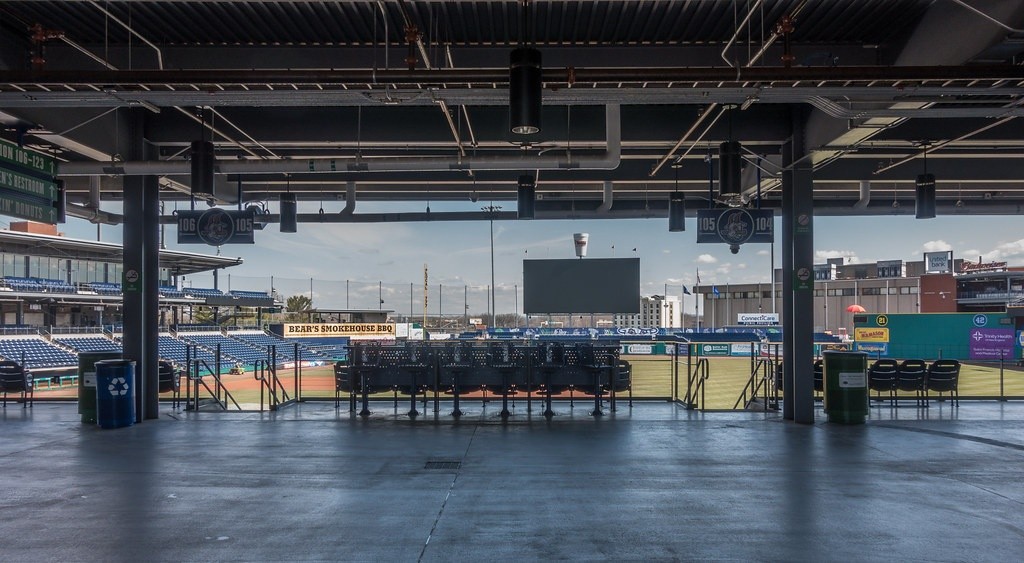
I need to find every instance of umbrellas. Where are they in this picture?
[844,304,866,313]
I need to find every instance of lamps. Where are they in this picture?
[718,103,741,197]
[914,143,935,219]
[191,100,214,197]
[510,1,541,134]
[280,173,297,233]
[516,169,535,219]
[670,164,685,233]
[245,199,270,230]
[51,178,66,224]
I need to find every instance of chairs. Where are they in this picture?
[0,361,35,407]
[334,343,633,421]
[775,358,963,411]
[158,361,181,408]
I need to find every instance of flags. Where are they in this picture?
[612,245,614,248]
[525,250,527,253]
[714,286,720,295]
[697,276,700,283]
[683,286,691,295]
[632,247,636,251]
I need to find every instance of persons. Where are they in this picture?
[79,311,96,327]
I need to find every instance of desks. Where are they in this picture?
[343,343,623,417]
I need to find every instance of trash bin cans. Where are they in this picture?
[822,350,870,423]
[93,359,137,428]
[77,349,122,423]
